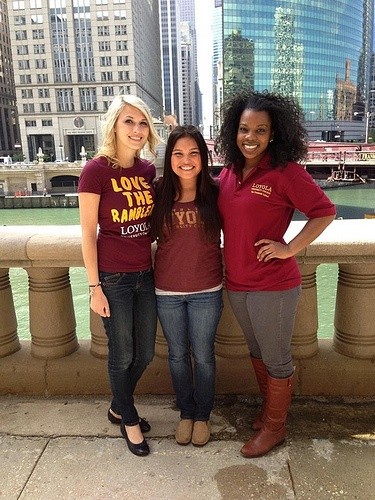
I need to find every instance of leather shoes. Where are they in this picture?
[121,421,150,455]
[108,408,151,433]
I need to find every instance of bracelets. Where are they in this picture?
[89,282,103,287]
[90,283,101,294]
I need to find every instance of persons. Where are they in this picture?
[77,95,337,459]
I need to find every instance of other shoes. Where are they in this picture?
[191,420,211,445]
[175,417,193,444]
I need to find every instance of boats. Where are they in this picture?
[318,170,367,189]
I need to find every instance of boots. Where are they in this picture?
[241,367,295,457]
[249,355,269,428]
